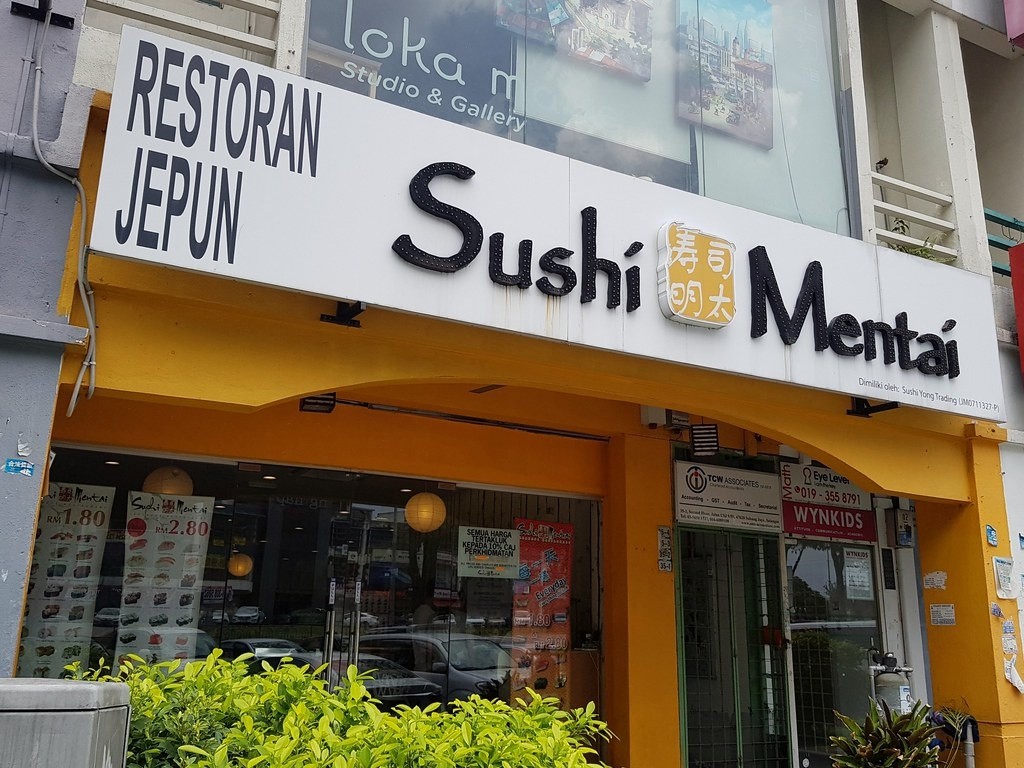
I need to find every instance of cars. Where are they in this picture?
[212,610,230,626]
[402,612,533,629]
[93,608,121,626]
[215,631,542,717]
[230,605,266,624]
[343,611,381,628]
[94,625,221,679]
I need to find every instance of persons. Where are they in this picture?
[408,577,466,673]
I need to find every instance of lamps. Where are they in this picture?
[689,415,719,457]
[300,391,336,413]
